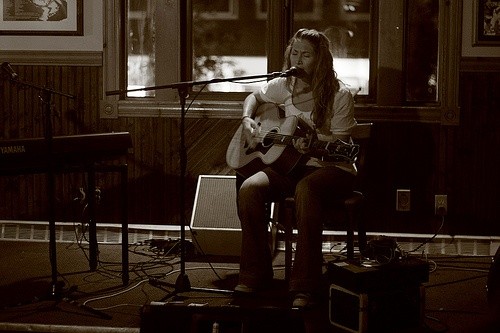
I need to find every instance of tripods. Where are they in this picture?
[0,76,113,321]
[105,71,284,303]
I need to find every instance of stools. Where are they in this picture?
[283,190,364,278]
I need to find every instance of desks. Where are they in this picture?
[0,163,129,297]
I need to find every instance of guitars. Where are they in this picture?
[225,101,360,180]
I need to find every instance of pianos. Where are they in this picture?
[0,131,132,173]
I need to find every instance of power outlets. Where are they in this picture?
[436,194,447,214]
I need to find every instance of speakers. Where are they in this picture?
[190,175,279,258]
[140,301,305,333]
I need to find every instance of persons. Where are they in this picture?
[233,28,359,310]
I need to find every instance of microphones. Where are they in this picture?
[280,67,298,77]
[0,62,18,78]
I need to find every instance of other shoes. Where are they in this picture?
[291,291,310,310]
[234,284,256,295]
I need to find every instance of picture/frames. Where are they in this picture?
[472,0,500,47]
[0,0,83,36]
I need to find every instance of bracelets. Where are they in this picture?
[240,115,250,122]
